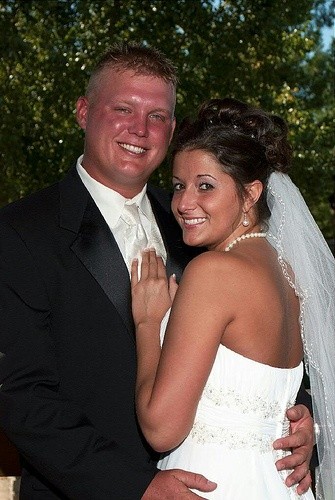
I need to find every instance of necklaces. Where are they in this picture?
[224,232,267,254]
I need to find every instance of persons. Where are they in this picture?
[130,96,322,500]
[1,41,324,500]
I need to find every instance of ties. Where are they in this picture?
[120,202,150,283]
